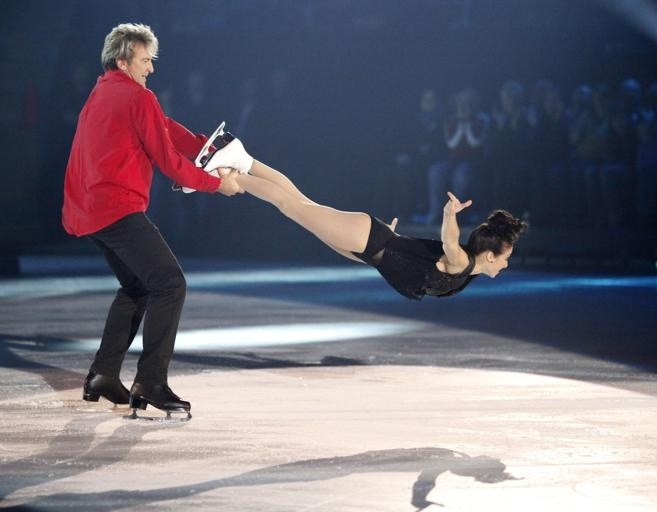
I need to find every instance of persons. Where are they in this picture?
[181,118,526,302]
[388,72,654,242]
[61,19,247,412]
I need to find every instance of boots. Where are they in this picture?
[180,167,232,194]
[199,132,254,175]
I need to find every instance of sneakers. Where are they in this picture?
[128,379,191,413]
[81,371,131,404]
